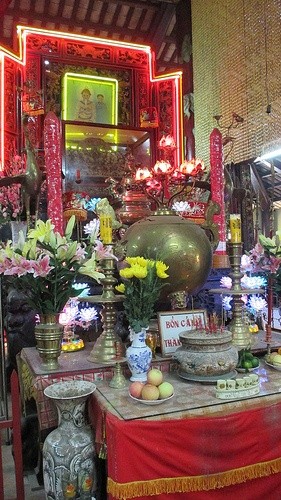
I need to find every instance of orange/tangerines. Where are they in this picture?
[240,350,259,368]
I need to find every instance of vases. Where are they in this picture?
[126,325,153,383]
[42,380,103,500]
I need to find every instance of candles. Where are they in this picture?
[210,129,228,257]
[115,341,120,361]
[266,324,271,343]
[43,109,63,240]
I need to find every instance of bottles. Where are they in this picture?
[40,380,98,500]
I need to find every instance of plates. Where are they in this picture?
[128,382,175,405]
[265,360,281,371]
[234,362,260,373]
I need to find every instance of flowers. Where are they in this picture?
[0,154,28,218]
[245,229,281,276]
[114,254,170,335]
[0,214,119,316]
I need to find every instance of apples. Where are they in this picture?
[269,346,281,364]
[142,383,159,400]
[147,369,163,386]
[129,381,145,398]
[157,381,173,399]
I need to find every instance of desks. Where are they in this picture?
[17,326,281,500]
